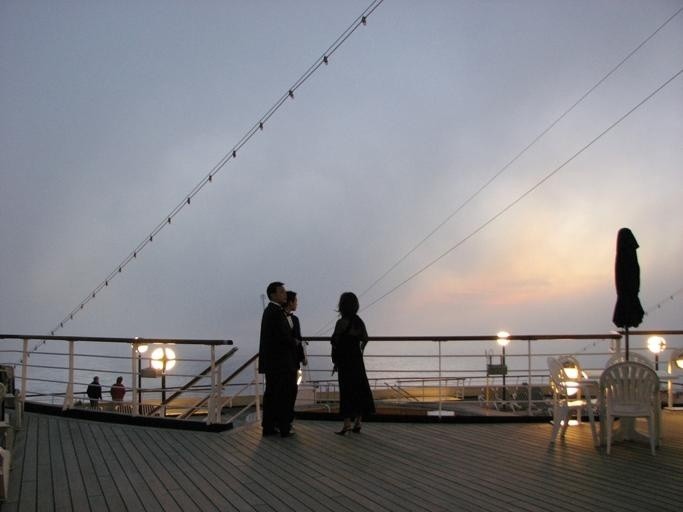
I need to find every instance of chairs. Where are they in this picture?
[546,351,663,457]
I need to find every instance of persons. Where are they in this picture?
[329,291,375,436]
[109,376,126,400]
[277,289,308,430]
[257,281,304,438]
[86,376,103,408]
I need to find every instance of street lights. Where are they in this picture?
[498,332,510,408]
[131,336,175,413]
[648,337,665,371]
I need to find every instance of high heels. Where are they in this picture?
[352,423,362,433]
[333,425,352,435]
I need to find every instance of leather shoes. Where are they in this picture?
[261,423,297,438]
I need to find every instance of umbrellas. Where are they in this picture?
[611,227,647,365]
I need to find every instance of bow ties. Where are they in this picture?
[286,312,293,317]
